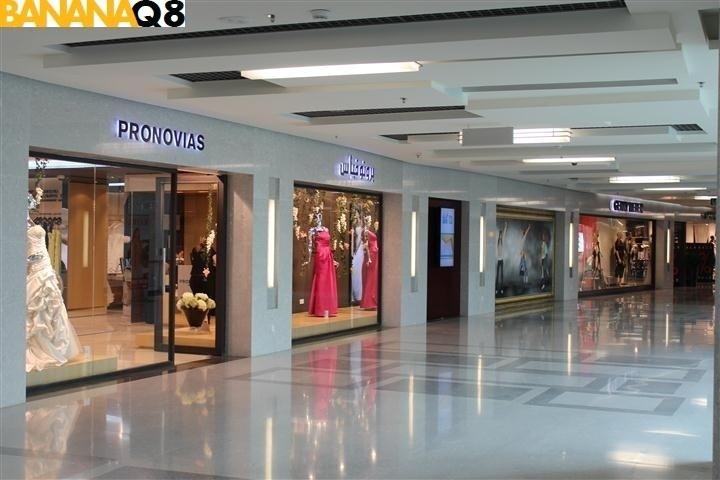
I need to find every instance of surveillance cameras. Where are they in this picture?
[572,162,577,166]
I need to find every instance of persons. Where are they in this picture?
[495,220,548,294]
[26,193,81,373]
[189,240,216,316]
[302,213,340,317]
[615,231,632,287]
[351,215,379,311]
[591,234,604,290]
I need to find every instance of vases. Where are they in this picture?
[183,306,209,327]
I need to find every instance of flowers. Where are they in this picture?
[176,292,216,313]
